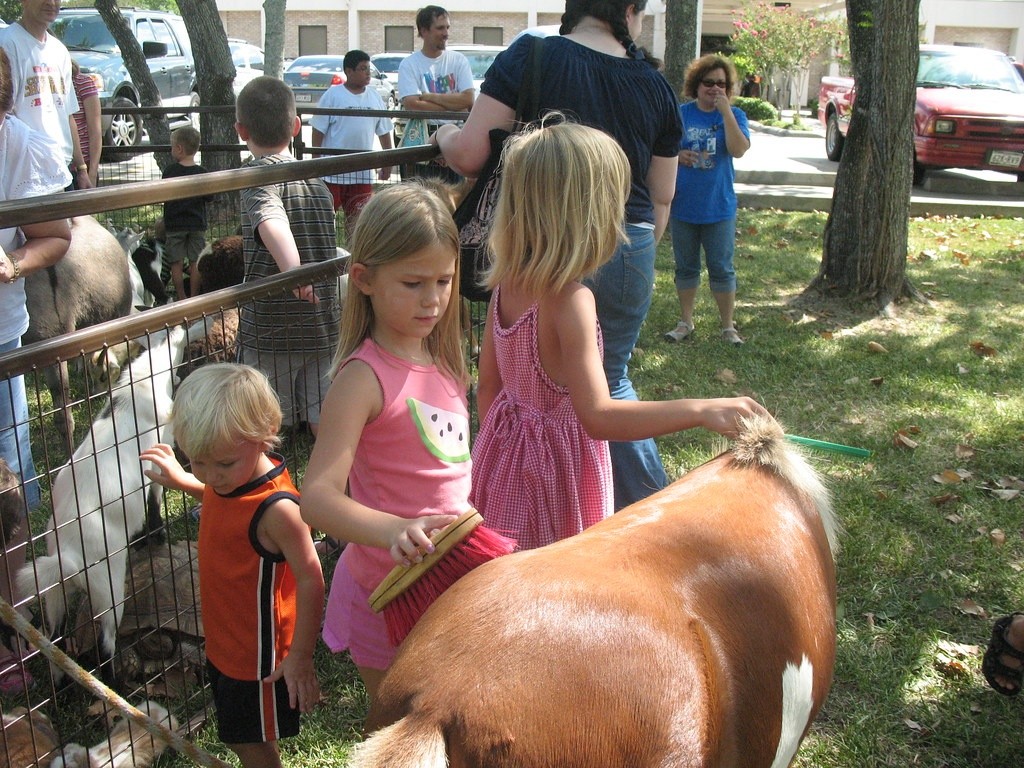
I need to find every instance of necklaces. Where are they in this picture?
[695,102,720,131]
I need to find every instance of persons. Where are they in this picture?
[666,54,750,347]
[308,50,393,251]
[982,612,1024,697]
[154,126,214,301]
[299,182,476,740]
[140,362,325,768]
[234,76,340,440]
[469,112,784,554]
[0,0,102,694]
[429,0,684,513]
[398,6,476,183]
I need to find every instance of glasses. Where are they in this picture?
[700,79,727,88]
[354,68,375,74]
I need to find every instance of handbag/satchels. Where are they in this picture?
[396,118,432,166]
[443,36,549,304]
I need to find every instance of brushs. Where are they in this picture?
[365,509,522,644]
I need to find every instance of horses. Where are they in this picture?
[342,435,842,768]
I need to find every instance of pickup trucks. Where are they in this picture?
[816,44,1024,189]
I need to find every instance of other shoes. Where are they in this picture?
[664,322,695,343]
[722,328,745,346]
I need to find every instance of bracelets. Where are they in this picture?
[72,164,87,172]
[435,129,438,144]
[4,253,19,285]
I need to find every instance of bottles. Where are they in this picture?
[686,128,705,168]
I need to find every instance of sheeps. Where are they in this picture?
[17,221,389,677]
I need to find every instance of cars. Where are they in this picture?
[226,40,508,125]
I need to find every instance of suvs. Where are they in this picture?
[46,5,201,166]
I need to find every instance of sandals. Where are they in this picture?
[981,612,1024,695]
